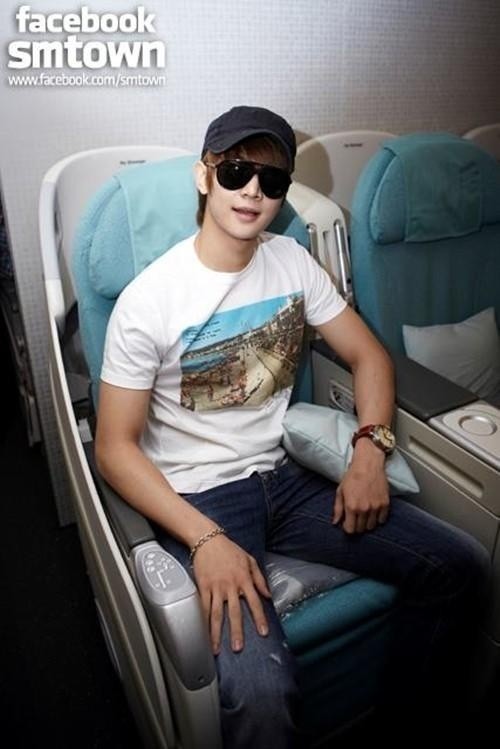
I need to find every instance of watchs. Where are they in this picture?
[351,422,396,458]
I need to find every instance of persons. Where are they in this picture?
[92,108,493,748]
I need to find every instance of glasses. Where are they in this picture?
[204,157,293,201]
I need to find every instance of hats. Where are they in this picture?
[203,105,297,170]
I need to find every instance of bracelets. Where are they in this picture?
[189,527,227,562]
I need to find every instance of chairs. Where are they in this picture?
[36,122,499,747]
